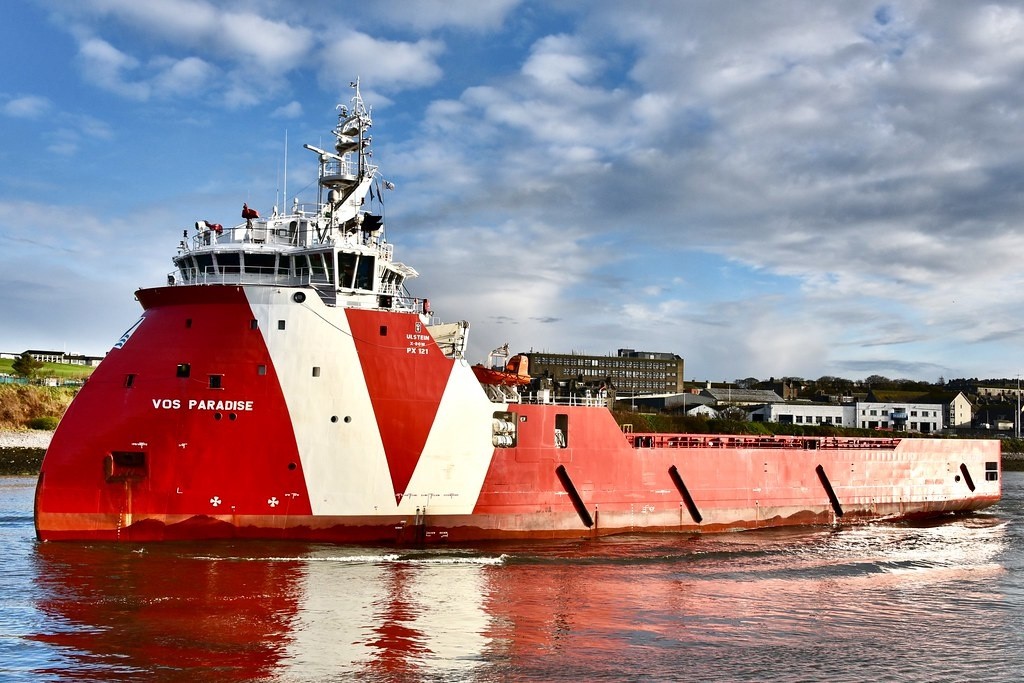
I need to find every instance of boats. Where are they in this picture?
[469,354,533,385]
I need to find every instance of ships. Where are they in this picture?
[33,76,1003,545]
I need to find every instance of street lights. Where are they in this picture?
[631,386,636,414]
